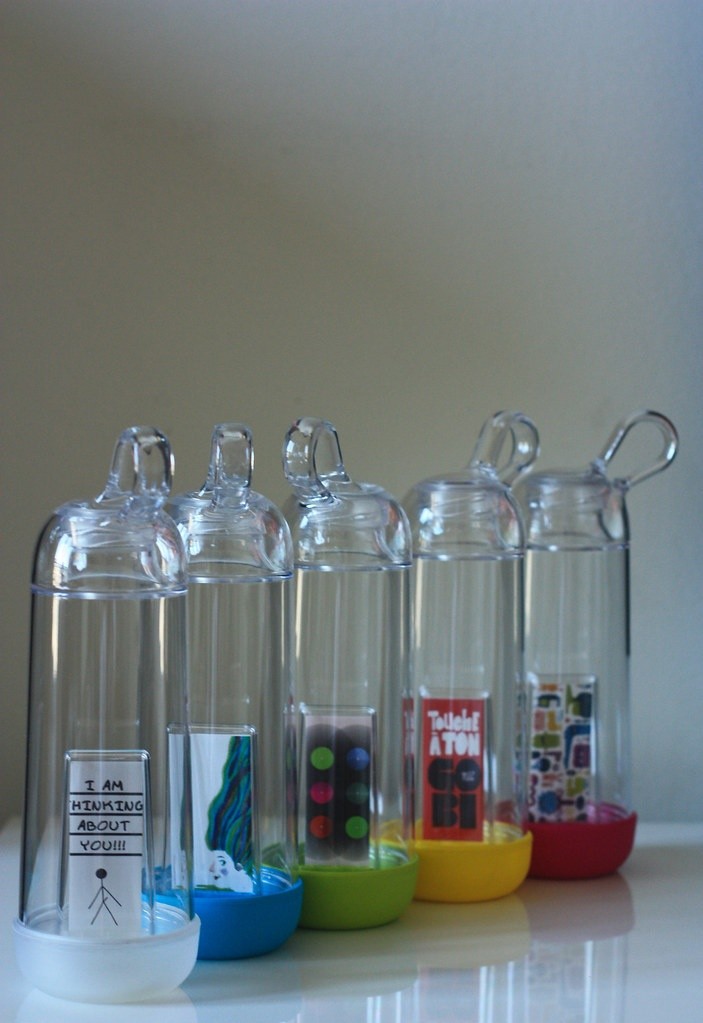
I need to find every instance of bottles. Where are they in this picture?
[143,417,304,964]
[255,417,423,937]
[376,409,543,906]
[7,419,206,1005]
[489,407,683,885]
